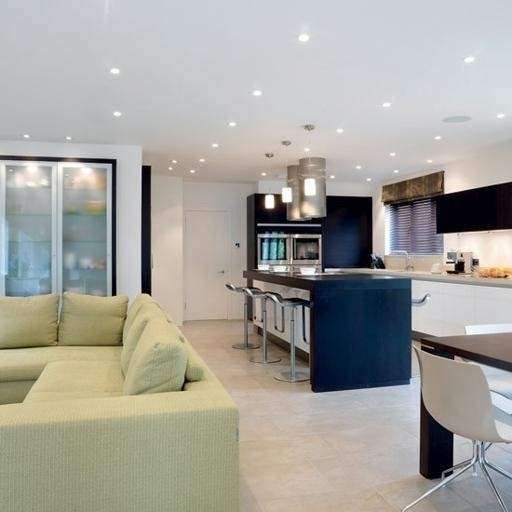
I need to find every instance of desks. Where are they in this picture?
[417,330,511,481]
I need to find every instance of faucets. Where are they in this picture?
[289,257,294,272]
[404,264,414,271]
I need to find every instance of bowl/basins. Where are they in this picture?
[258,265,269,269]
[85,199,104,215]
[79,257,93,268]
[273,266,286,271]
[299,267,315,274]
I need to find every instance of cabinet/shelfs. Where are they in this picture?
[410,277,512,346]
[435,182,512,234]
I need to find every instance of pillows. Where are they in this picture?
[1,291,60,351]
[56,289,130,347]
[120,290,190,403]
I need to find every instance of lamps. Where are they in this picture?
[263,152,276,210]
[302,124,318,198]
[279,139,293,205]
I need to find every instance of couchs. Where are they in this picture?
[1,293,241,510]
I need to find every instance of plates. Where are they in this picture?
[296,274,318,277]
[270,270,289,274]
[252,268,270,272]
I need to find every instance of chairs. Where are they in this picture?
[224,283,262,349]
[263,290,316,383]
[242,287,285,365]
[399,344,511,512]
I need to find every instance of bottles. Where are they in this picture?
[262,239,270,263]
[457,253,464,271]
[270,239,277,260]
[277,238,286,259]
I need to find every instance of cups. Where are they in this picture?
[431,263,441,274]
